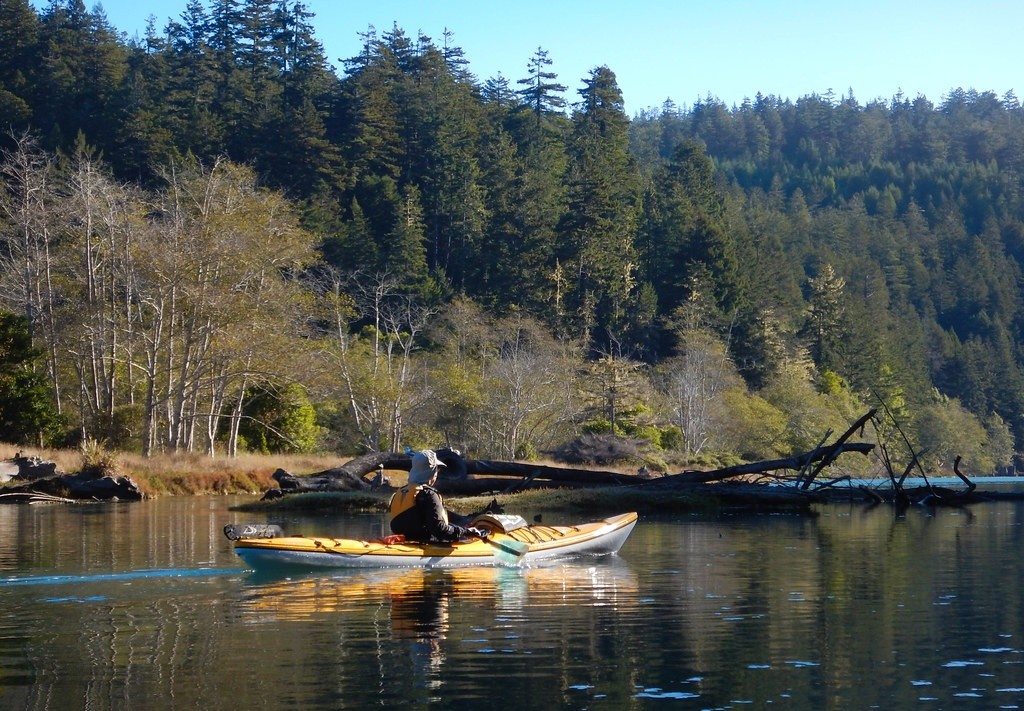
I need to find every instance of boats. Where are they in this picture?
[230,511,640,583]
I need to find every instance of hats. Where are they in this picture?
[408,450,447,483]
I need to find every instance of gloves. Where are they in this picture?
[467,526,481,537]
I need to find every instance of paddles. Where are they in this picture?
[447,520,530,565]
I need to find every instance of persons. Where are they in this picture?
[389,450,535,546]
